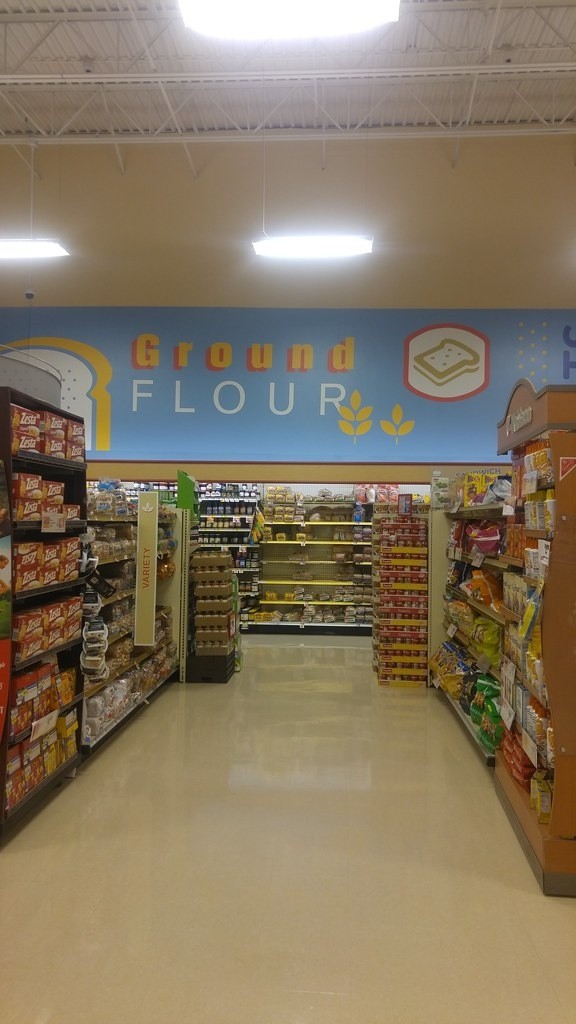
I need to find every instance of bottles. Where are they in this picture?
[526,696,555,768]
[524,537,540,579]
[524,489,554,539]
[524,441,552,483]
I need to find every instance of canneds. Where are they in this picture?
[198,483,258,497]
[378,517,428,682]
[200,519,235,528]
[153,483,177,490]
[198,534,248,543]
[194,550,233,647]
[239,575,260,592]
[126,482,149,496]
[233,551,260,568]
[241,595,261,621]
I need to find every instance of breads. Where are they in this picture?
[26,426,85,651]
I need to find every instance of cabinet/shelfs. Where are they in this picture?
[0,384,87,823]
[353,501,431,637]
[433,500,508,768]
[63,489,189,759]
[183,509,201,682]
[254,498,353,640]
[496,376,576,898]
[189,496,265,634]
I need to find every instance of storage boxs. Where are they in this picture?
[5,402,85,811]
